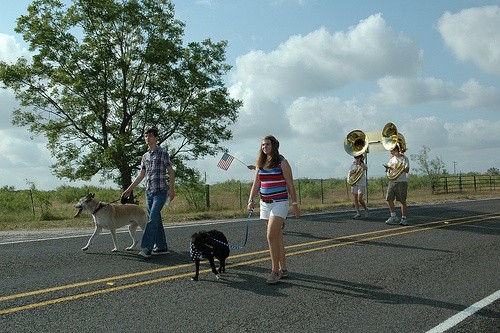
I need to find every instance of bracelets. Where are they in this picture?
[292,202,297,205]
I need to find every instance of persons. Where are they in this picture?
[349,155,370,219]
[247,135,300,283]
[122,129,175,257]
[385,145,410,225]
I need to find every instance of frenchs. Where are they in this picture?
[381,122,409,181]
[343,129,381,185]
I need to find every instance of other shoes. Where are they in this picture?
[138,248,152,257]
[151,246,171,254]
[365,208,370,217]
[399,217,407,225]
[265,268,289,284]
[353,213,361,219]
[385,216,399,224]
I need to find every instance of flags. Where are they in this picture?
[217,153,234,171]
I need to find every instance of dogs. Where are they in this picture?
[190,230,230,281]
[74,193,148,252]
